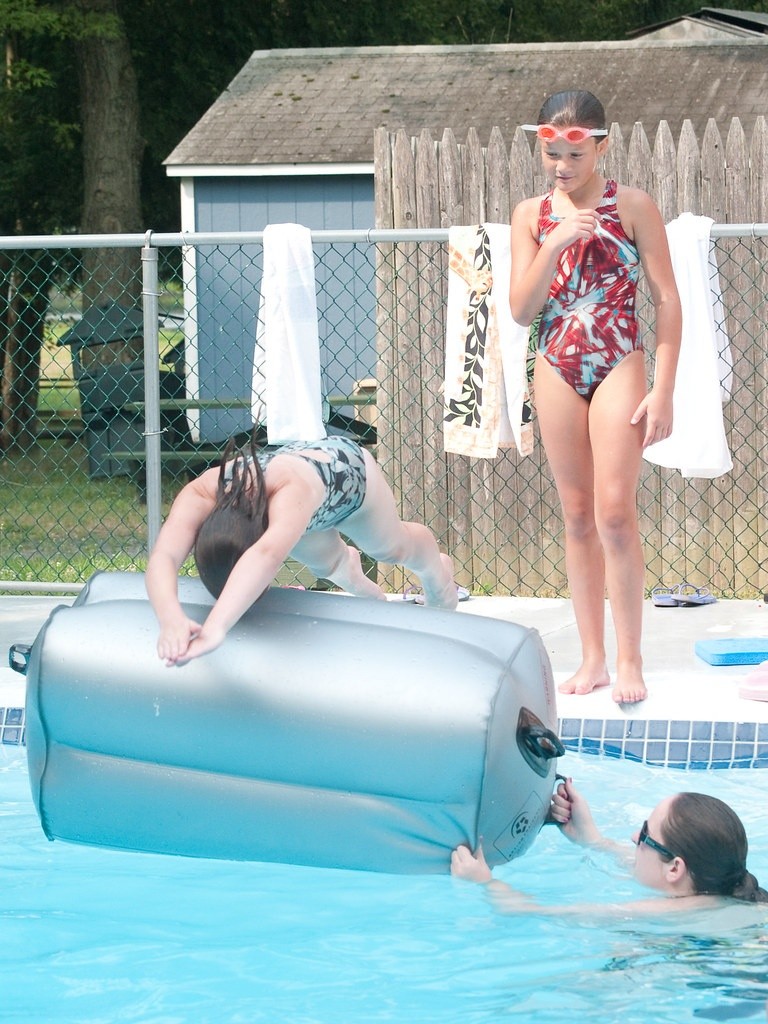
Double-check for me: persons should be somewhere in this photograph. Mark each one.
[510,89,682,705]
[146,438,459,666]
[452,776,768,916]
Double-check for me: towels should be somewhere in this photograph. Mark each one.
[250,211,735,480]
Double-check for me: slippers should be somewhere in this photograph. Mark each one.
[390,586,422,603]
[416,582,470,605]
[652,585,679,606]
[671,584,717,604]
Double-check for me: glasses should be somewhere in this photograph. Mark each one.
[537,124,608,144]
[638,820,676,861]
